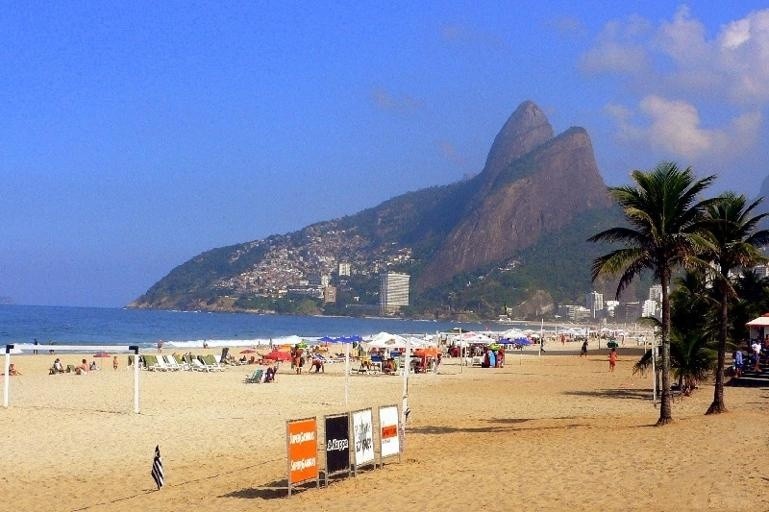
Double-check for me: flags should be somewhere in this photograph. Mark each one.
[151,445,164,490]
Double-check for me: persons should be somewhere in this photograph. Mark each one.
[9,363,18,376]
[203,339,207,348]
[607,348,618,371]
[77,358,89,374]
[53,356,61,371]
[732,333,769,379]
[223,343,504,384]
[34,338,39,354]
[182,352,188,364]
[56,358,64,369]
[157,340,163,353]
[113,356,118,369]
[47,337,56,354]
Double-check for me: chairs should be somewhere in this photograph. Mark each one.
[129,350,225,373]
[242,365,278,383]
